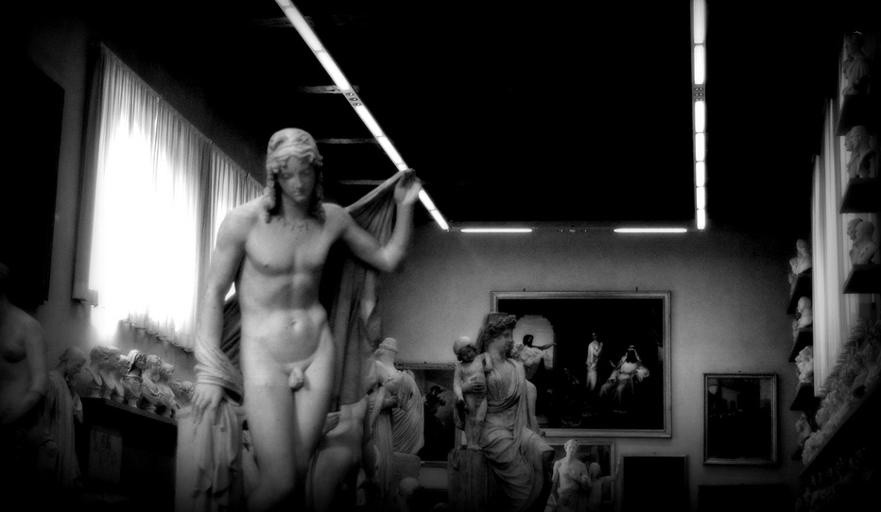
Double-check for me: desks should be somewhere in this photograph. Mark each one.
[80,397,176,512]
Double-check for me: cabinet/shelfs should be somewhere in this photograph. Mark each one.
[835,94,879,293]
[787,268,813,459]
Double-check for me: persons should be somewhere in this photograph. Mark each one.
[190,125,421,512]
[787,40,881,467]
[0,261,649,512]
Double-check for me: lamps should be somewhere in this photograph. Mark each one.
[691,0,708,229]
[449,225,688,234]
[275,0,450,234]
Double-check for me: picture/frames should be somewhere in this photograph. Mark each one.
[702,373,778,467]
[548,442,616,512]
[491,291,672,438]
[393,362,462,468]
[619,453,690,512]
